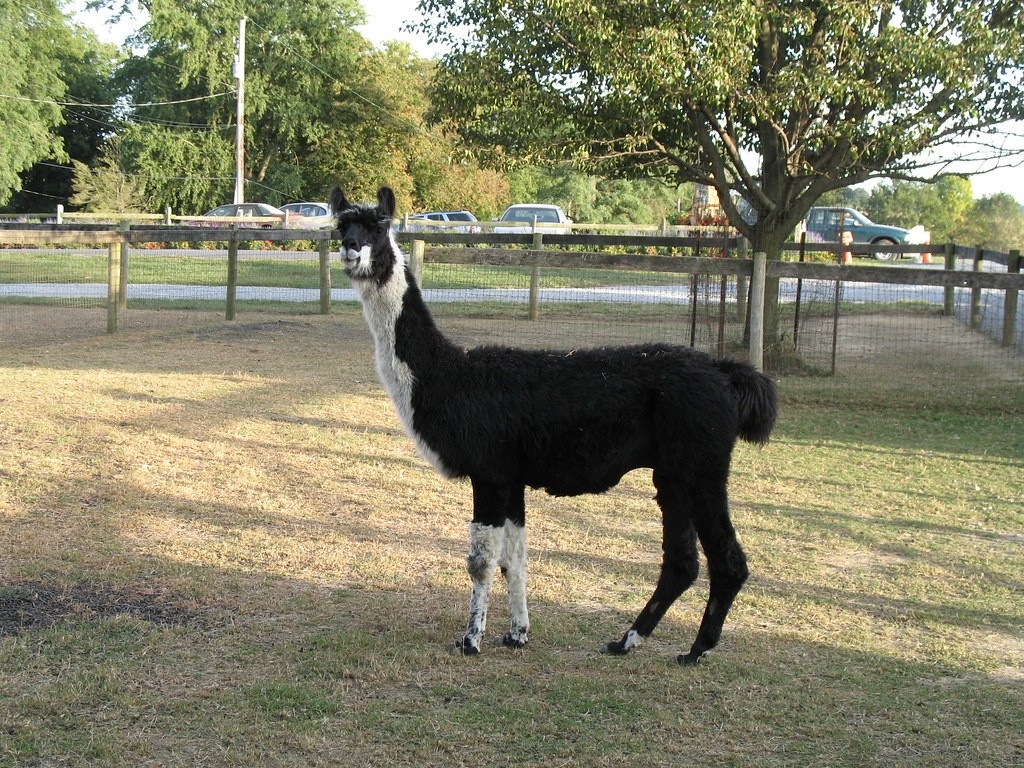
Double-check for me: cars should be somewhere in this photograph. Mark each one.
[491,203,572,234]
[275,203,338,231]
[184,201,305,245]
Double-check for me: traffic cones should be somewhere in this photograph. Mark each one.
[922,241,935,266]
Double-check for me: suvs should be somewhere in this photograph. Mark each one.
[400,209,483,234]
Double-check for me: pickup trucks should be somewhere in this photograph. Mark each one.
[804,206,913,263]
